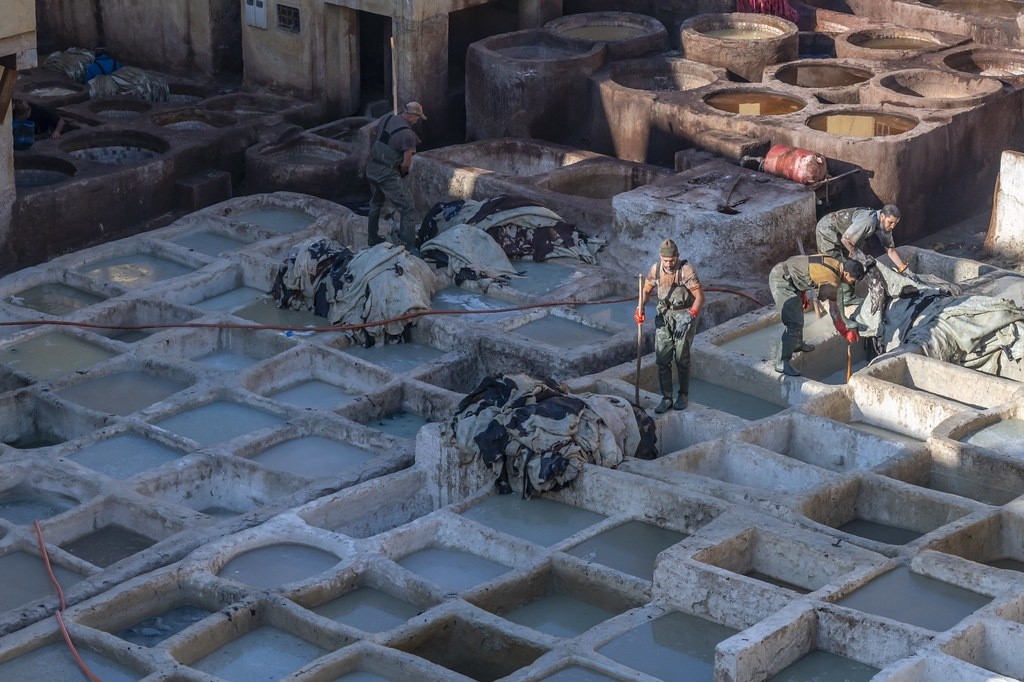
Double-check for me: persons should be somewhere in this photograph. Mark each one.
[13,102,65,140]
[634,239,704,414]
[768,254,864,376]
[816,205,909,328]
[363,101,427,260]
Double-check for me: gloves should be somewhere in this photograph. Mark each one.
[673,312,695,340]
[800,290,810,309]
[833,319,859,343]
[634,307,645,323]
[899,265,910,277]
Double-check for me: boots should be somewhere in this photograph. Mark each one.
[400,221,418,256]
[368,214,386,246]
[655,369,673,414]
[775,331,801,376]
[673,367,690,410]
[793,327,815,352]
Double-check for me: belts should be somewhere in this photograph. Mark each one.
[371,159,394,169]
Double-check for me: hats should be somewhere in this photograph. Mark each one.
[403,102,427,120]
[658,239,678,258]
